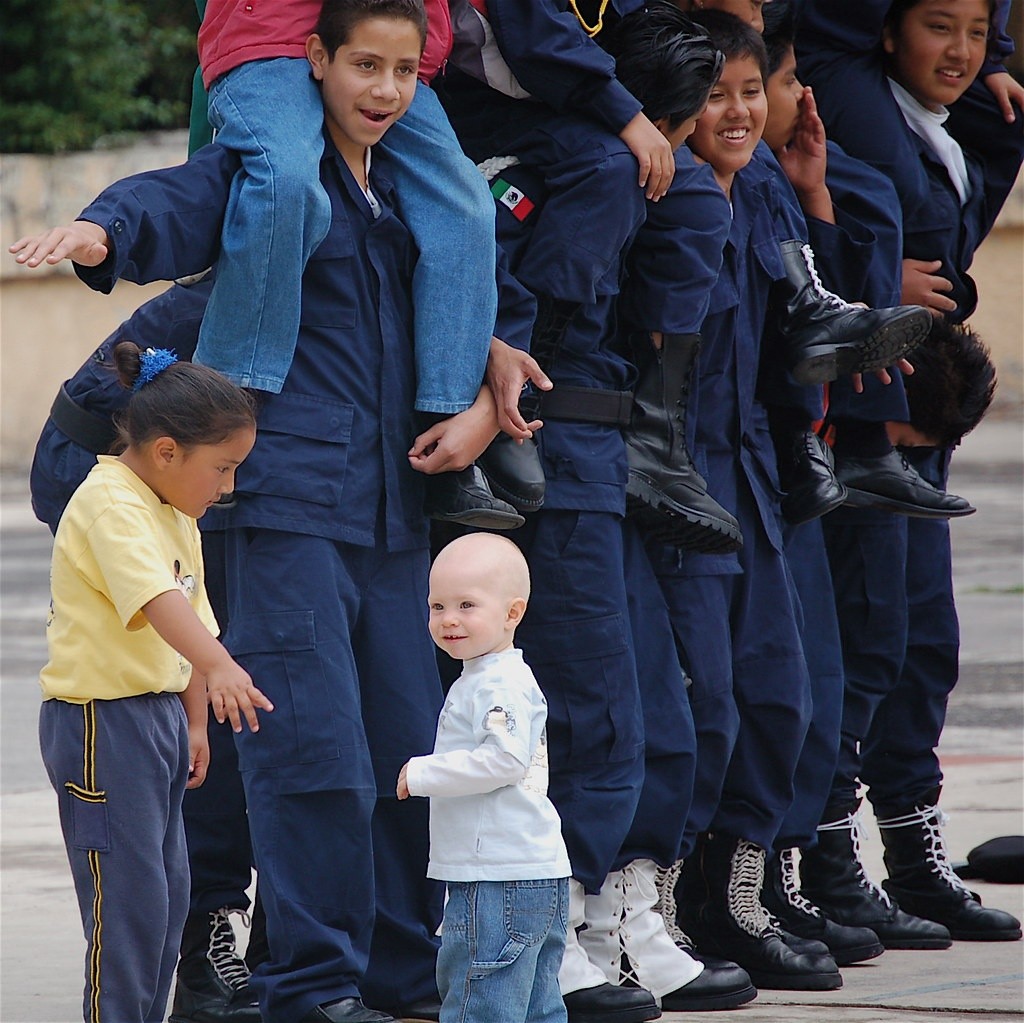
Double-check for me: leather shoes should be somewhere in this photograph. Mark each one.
[562,983,660,1023]
[299,997,401,1023]
[776,429,848,527]
[383,999,442,1022]
[661,967,757,1011]
[834,447,976,518]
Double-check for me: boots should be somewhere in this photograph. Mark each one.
[875,786,1022,940]
[624,333,742,555]
[758,841,884,965]
[696,832,842,989]
[211,388,270,508]
[168,905,265,1023]
[425,413,524,529]
[655,859,739,969]
[753,888,836,966]
[766,239,933,385]
[478,285,584,513]
[800,797,952,951]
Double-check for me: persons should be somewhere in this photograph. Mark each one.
[394,531,572,1023]
[7,0,1024,1023]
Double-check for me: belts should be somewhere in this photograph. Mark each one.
[50,379,129,456]
[539,383,633,425]
